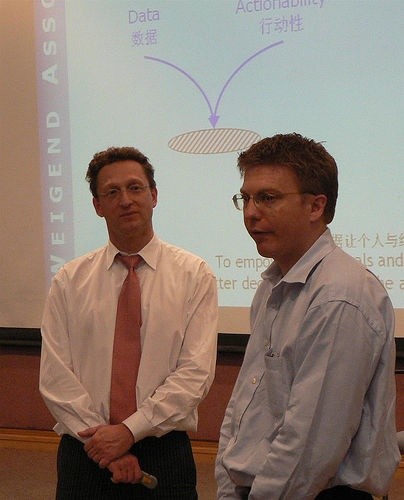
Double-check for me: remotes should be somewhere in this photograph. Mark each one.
[140,470,158,489]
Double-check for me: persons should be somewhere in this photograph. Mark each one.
[39,146,218,500]
[214,131,402,500]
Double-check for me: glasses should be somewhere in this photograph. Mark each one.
[231,191,318,210]
[96,183,154,201]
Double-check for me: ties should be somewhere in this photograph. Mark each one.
[108,255,147,426]
